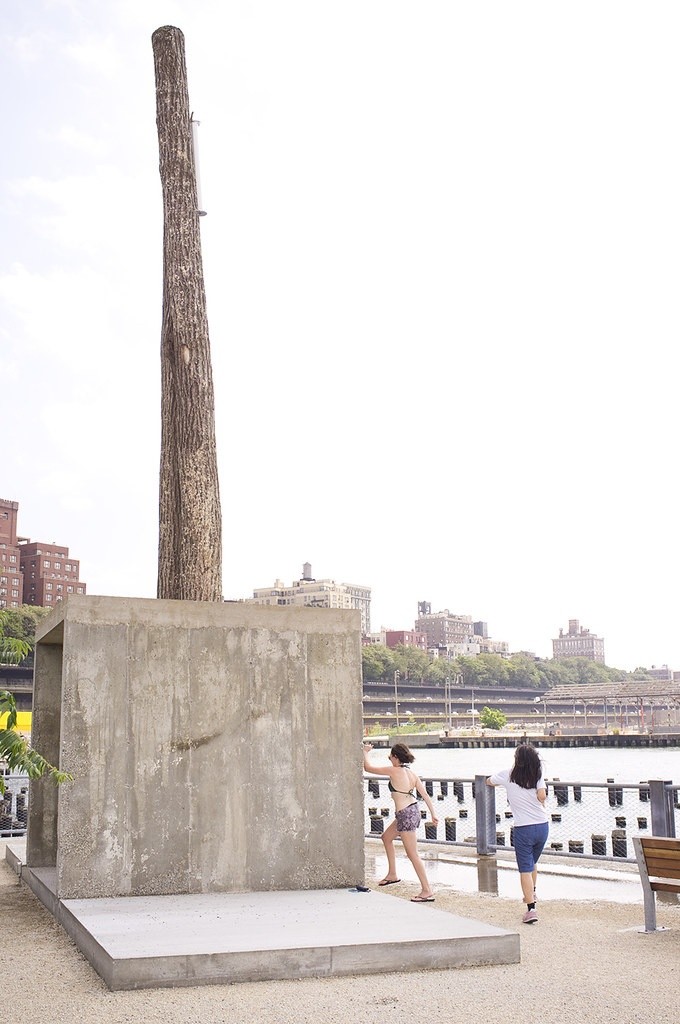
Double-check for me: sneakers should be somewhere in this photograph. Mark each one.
[522,908,539,922]
[523,892,537,903]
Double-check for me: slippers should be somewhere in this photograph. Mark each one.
[378,879,401,886]
[411,895,435,902]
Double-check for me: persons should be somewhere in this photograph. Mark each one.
[486,745,549,922]
[0,784,29,837]
[363,743,438,902]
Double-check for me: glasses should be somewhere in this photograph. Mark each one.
[388,757,393,760]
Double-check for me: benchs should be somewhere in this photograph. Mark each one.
[632,835,680,933]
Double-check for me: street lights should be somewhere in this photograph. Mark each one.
[395,670,400,726]
[446,677,452,725]
